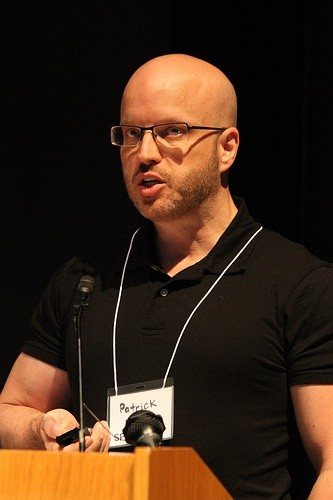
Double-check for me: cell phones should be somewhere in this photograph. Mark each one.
[56,426,84,448]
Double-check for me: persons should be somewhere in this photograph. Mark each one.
[0,54,333,500]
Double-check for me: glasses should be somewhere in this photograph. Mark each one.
[111,121,223,150]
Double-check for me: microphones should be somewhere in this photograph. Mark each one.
[75,277,88,452]
[123,409,166,448]
